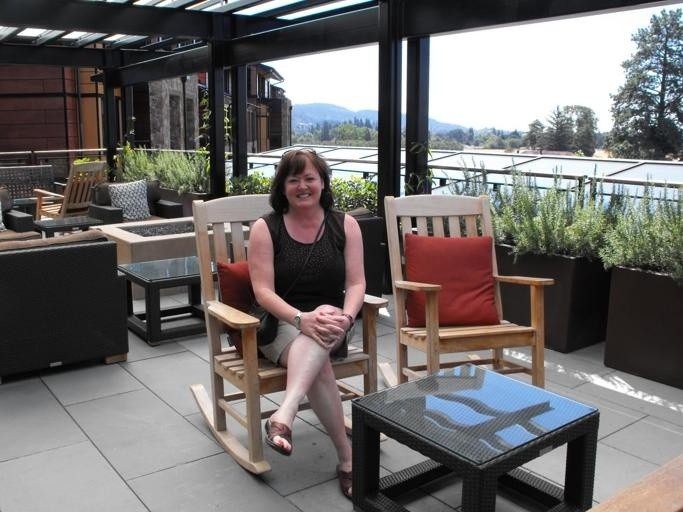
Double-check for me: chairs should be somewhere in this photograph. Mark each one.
[0,187,41,242]
[384,194,555,389]
[0,230,129,383]
[32,161,106,236]
[89,180,183,225]
[190,193,388,475]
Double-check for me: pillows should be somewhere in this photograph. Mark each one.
[58,177,95,213]
[405,233,500,328]
[107,179,152,223]
[217,262,255,359]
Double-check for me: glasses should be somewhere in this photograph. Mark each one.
[281,148,317,162]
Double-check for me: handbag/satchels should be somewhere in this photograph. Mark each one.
[239,303,277,347]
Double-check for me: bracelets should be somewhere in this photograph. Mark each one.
[341,313,353,328]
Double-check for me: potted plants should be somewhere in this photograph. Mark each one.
[599,173,683,391]
[496,157,625,354]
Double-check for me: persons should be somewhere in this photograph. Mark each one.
[239,148,366,498]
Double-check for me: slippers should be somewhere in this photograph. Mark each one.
[336,464,352,500]
[265,419,293,456]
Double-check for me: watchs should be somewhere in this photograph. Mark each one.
[294,311,301,330]
